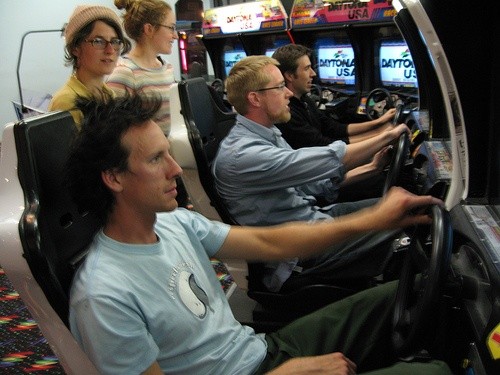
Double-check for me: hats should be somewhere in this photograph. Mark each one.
[66,4,124,56]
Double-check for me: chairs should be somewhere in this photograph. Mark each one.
[0,108,101,375]
[178,77,294,308]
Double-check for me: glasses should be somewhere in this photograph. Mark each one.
[82,36,121,49]
[157,24,174,32]
[255,83,286,91]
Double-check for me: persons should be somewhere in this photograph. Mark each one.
[49,4,132,135]
[105,0,195,212]
[68,86,451,375]
[213,43,413,293]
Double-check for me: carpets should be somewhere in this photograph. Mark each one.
[1,191,237,375]
